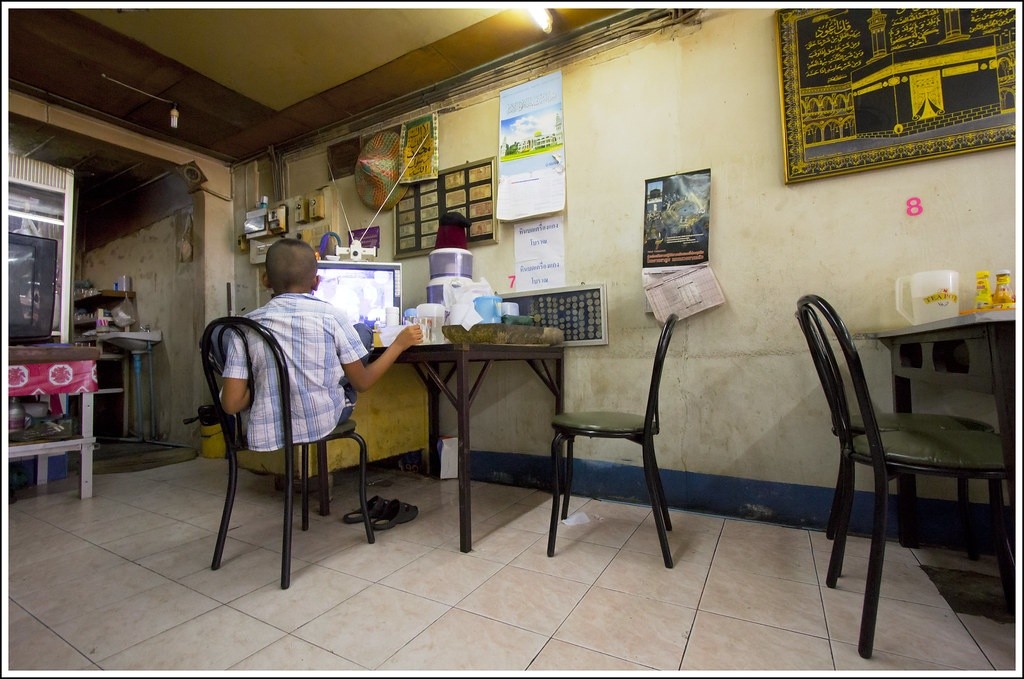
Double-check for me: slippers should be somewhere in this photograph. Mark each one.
[370,498,418,530]
[344,496,392,523]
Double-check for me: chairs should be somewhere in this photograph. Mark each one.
[795,294,1015,660]
[199,316,374,590]
[546,314,679,569]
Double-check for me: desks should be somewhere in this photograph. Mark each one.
[316,343,564,552]
[851,307,1015,549]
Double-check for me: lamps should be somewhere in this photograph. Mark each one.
[528,8,553,35]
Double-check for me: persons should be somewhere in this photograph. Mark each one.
[221,238,423,452]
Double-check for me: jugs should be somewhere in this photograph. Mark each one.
[896,269,959,325]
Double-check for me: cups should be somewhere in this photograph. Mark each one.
[386,306,400,327]
[403,308,416,325]
[473,296,503,324]
[416,303,446,344]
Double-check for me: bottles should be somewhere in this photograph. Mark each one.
[991,270,1016,308]
[975,270,994,310]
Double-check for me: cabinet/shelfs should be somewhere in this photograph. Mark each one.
[73,290,136,439]
[9,346,102,500]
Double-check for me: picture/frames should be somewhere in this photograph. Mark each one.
[391,156,501,262]
[498,282,609,347]
[775,8,1015,185]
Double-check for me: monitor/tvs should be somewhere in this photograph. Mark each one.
[313,261,402,329]
[7,232,58,346]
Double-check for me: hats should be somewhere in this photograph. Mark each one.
[354,131,409,211]
